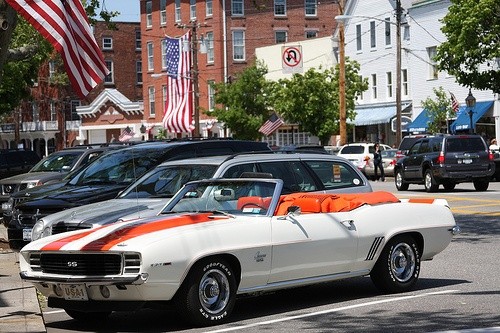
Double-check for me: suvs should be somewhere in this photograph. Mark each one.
[276,142,333,183]
[0,139,147,228]
[7,135,277,252]
[338,142,391,174]
[396,134,435,161]
[392,132,497,192]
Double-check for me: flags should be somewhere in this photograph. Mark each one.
[6,0,112,101]
[161,29,194,133]
[257,111,283,136]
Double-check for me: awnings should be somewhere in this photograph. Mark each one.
[406,100,494,132]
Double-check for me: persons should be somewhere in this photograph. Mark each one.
[489,138,500,156]
[374,142,386,183]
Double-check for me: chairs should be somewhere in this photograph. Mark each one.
[292,197,322,214]
[237,197,264,210]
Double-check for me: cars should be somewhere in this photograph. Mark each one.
[30,151,375,242]
[324,145,344,157]
[363,148,401,181]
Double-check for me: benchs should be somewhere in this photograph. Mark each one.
[0,151,34,171]
[276,195,361,215]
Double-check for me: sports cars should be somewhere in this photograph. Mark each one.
[16,170,461,328]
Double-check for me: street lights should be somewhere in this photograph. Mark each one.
[150,71,200,137]
[333,13,402,150]
